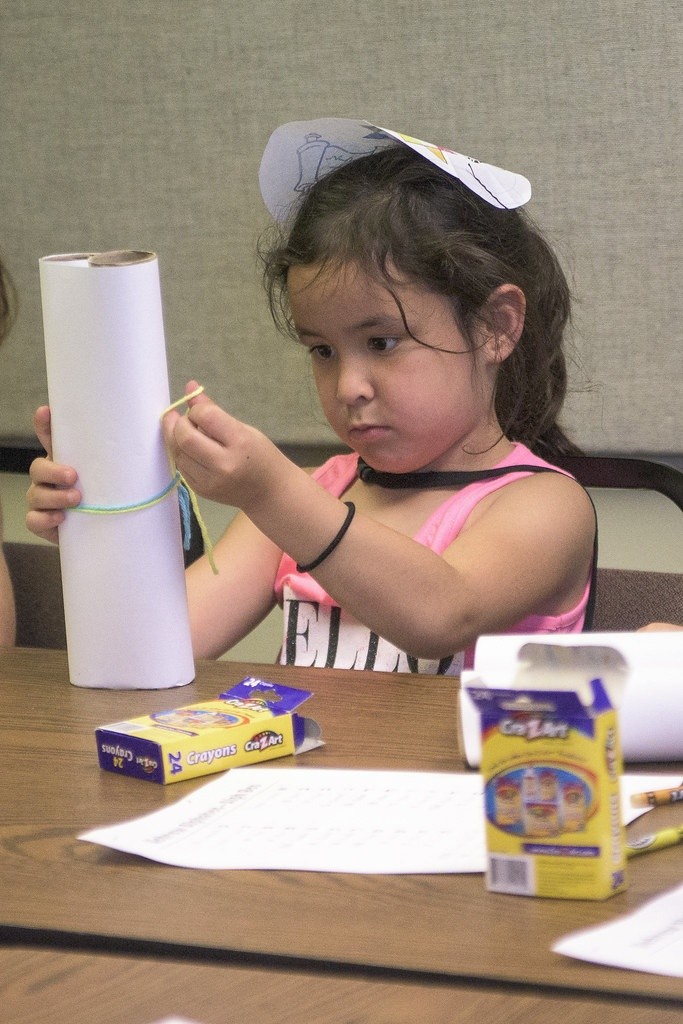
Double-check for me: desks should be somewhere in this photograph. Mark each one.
[0,658,683,1024]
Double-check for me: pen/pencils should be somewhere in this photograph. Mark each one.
[625,827,683,858]
[631,785,683,808]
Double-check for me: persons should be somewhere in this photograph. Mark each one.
[0,258,20,645]
[30,117,597,677]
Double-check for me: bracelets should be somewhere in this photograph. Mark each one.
[297,501,355,573]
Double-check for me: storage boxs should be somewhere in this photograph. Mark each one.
[95,676,325,787]
[464,640,627,899]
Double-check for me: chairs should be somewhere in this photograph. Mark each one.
[571,456,683,635]
